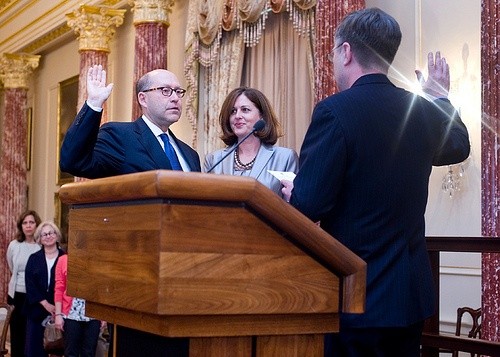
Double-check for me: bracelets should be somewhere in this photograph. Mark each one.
[53,313,64,317]
[48,307,56,314]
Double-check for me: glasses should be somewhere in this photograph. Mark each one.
[40,231,57,238]
[141,86,187,100]
[327,42,344,64]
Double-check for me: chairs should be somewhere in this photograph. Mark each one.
[0,303,15,357]
[439,306,482,357]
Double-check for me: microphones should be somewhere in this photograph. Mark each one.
[206,119,266,173]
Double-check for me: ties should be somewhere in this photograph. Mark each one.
[156,132,183,170]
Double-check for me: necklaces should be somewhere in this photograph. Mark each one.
[234,149,256,171]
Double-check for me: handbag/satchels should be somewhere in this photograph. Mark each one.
[40,313,69,352]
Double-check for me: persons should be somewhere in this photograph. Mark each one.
[203,87,299,203]
[25,223,64,357]
[5,210,43,357]
[54,253,101,357]
[281,7,471,357]
[60,64,201,357]
